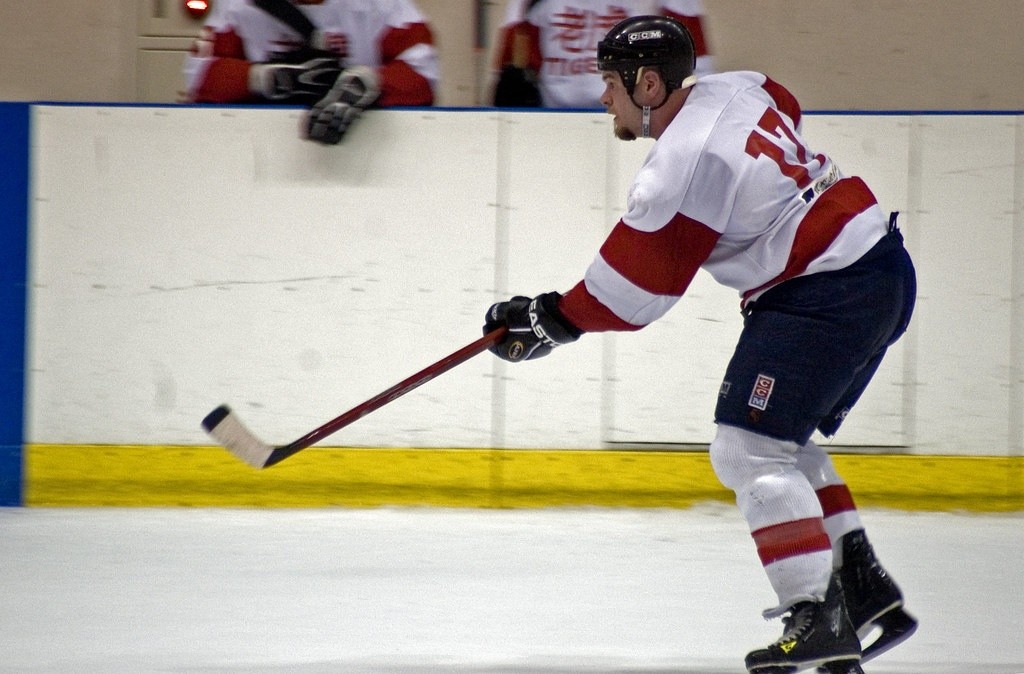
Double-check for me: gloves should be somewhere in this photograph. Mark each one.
[483,290,586,362]
[306,74,368,144]
[255,56,341,97]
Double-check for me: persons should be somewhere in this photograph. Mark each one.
[486,0,713,109]
[483,15,916,674]
[175,0,440,146]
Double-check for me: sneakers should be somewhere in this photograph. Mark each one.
[833,527,919,664]
[746,571,863,674]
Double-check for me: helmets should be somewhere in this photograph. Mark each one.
[596,15,695,88]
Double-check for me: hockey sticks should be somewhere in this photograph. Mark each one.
[200,324,509,468]
